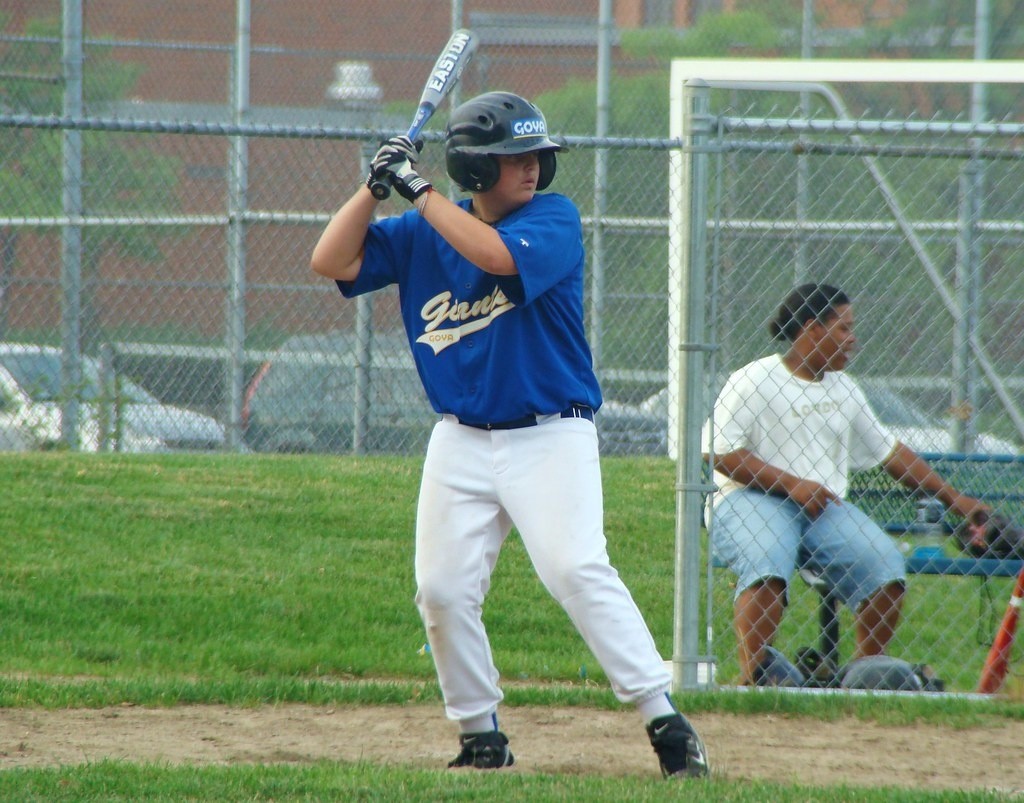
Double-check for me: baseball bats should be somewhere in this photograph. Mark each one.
[976,568,1024,692]
[369,28,481,201]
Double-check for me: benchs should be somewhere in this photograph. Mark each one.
[708,456,1024,672]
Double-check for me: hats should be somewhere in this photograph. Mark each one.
[446,90,571,192]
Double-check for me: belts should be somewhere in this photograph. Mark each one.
[459,402,594,431]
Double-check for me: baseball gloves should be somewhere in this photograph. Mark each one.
[955,507,1023,558]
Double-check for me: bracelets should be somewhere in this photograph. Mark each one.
[419,189,437,215]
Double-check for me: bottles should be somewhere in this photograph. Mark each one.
[913,488,945,560]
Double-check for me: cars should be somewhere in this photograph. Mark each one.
[640,370,1020,454]
[0,343,241,455]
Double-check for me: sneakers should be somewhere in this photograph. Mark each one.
[646,711,708,779]
[447,731,515,769]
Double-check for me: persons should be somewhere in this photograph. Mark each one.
[700,285,992,685]
[311,89,710,778]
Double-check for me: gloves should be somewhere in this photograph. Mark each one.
[365,135,432,202]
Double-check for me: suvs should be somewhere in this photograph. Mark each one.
[240,331,668,455]
